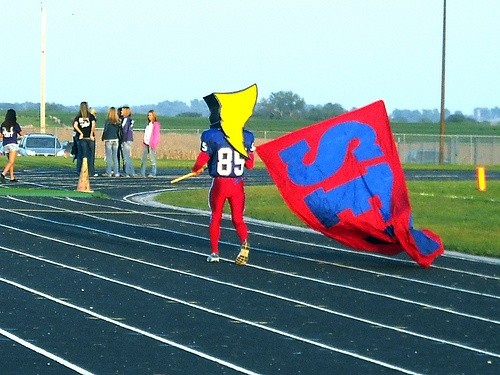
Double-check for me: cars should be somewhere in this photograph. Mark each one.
[0,142,21,156]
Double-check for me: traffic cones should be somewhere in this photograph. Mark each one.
[74,157,94,193]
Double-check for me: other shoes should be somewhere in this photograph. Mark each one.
[10,179,18,182]
[101,173,111,177]
[113,174,119,177]
[207,253,219,262]
[236,242,249,266]
[0,174,6,183]
[94,173,98,176]
[148,175,156,177]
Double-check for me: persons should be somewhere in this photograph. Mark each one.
[101,106,134,178]
[0,109,26,184]
[72,102,99,177]
[192,83,258,266]
[136,109,160,178]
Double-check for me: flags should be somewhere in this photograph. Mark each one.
[256,100,444,268]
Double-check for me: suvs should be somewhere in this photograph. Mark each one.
[62,141,76,158]
[19,132,67,158]
